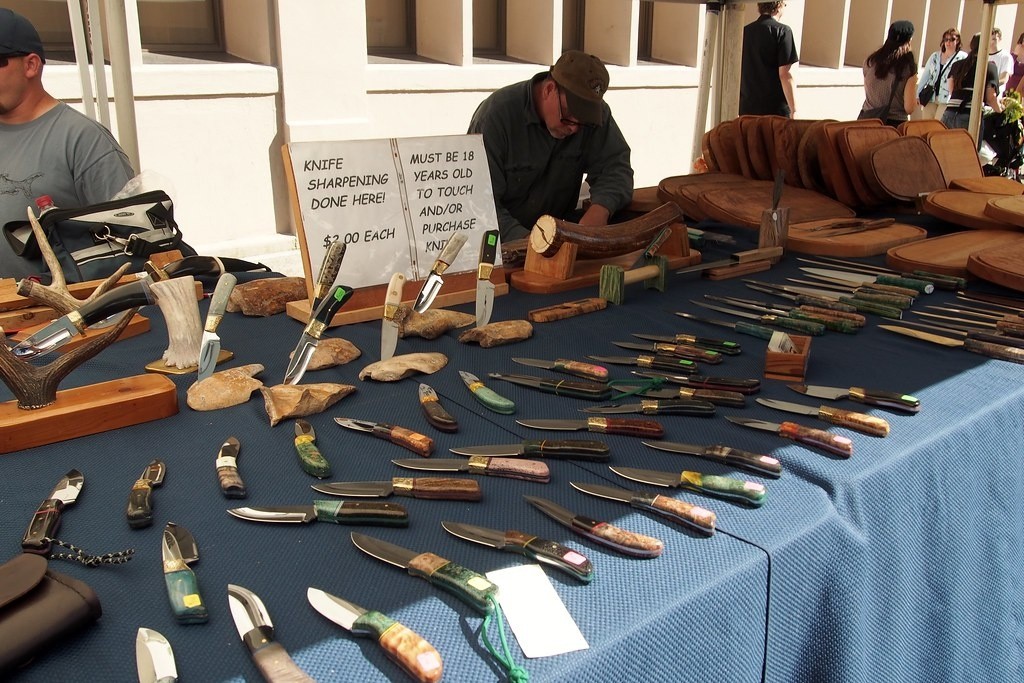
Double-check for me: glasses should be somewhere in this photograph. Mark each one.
[943,38,957,42]
[0,53,45,67]
[555,82,596,128]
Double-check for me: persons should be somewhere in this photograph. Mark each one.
[941,31,1003,132]
[738,0,801,121]
[916,27,968,121]
[0,8,137,285]
[987,27,1014,99]
[468,50,635,247]
[857,21,921,129]
[1002,33,1024,185]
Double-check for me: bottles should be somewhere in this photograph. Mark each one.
[34,194,59,220]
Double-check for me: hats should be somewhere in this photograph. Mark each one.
[0,8,45,61]
[551,50,609,128]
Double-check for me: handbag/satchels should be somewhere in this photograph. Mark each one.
[857,106,890,126]
[3,190,197,288]
[919,85,934,106]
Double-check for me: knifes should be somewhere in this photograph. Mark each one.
[9,218,1023,682]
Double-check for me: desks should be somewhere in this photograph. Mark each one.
[0,254,1023,683]
